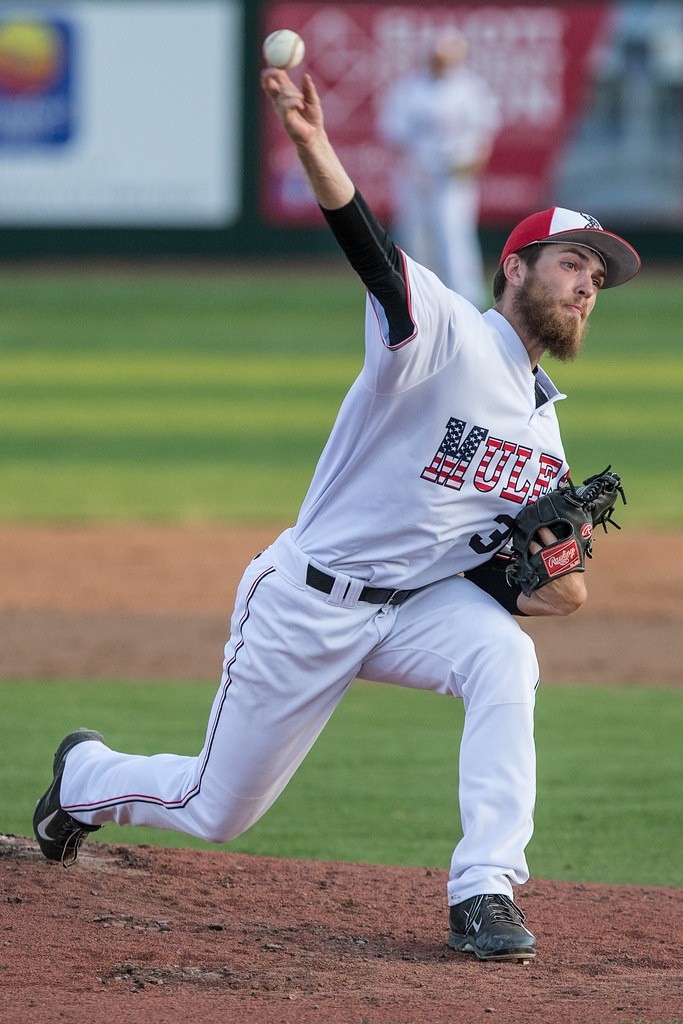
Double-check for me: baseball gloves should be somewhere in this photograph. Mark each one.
[508,470,620,599]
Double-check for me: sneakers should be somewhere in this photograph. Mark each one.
[33,730,105,862]
[448,894,539,959]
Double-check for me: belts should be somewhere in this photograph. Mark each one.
[306,562,412,605]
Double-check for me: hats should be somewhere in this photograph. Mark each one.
[499,206,640,289]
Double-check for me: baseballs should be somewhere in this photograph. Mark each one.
[262,29,306,70]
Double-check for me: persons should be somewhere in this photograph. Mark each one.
[381,28,500,311]
[31,68,640,965]
